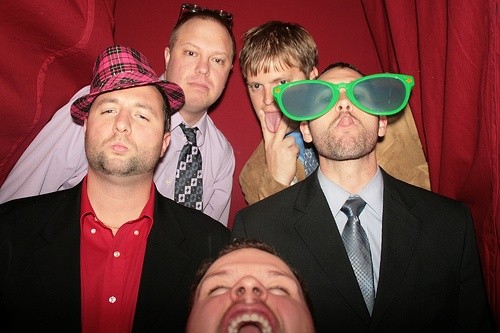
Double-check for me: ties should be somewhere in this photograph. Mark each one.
[304,143,320,177]
[174,122,203,212]
[340,197,375,316]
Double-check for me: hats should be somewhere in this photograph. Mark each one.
[70,45,186,126]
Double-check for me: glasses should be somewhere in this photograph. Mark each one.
[272,73,414,120]
[178,2,233,29]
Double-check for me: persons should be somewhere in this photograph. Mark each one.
[0,11,236,228]
[231,63,488,333]
[238,20,431,205]
[0,45,232,333]
[185,236,316,333]
